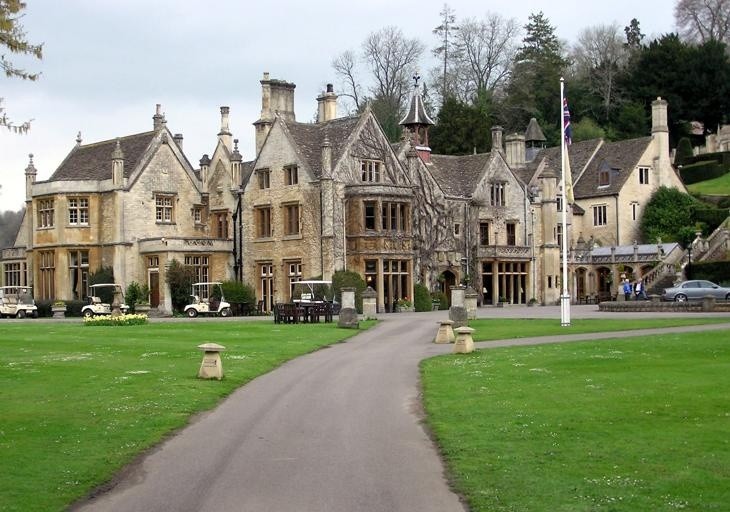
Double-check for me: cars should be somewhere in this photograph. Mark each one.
[662,280,730,302]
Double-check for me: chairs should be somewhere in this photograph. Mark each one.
[272,299,333,324]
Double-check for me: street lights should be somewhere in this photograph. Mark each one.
[686,237,692,270]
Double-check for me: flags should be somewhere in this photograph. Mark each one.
[565,141,577,206]
[564,95,576,145]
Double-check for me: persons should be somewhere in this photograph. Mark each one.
[482,284,490,304]
[635,278,651,300]
[623,278,634,299]
